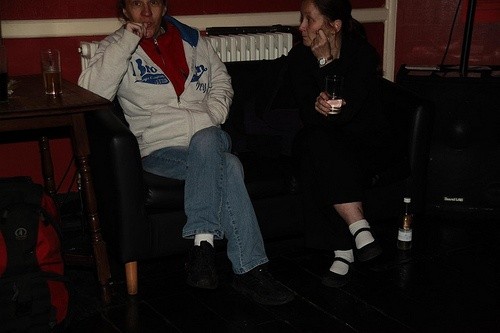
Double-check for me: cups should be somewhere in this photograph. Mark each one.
[41,47,63,95]
[325,74,343,114]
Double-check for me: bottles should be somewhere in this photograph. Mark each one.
[397,198,415,250]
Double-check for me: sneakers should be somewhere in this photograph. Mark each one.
[184,241,220,289]
[235,265,295,304]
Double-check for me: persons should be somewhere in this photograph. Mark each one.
[74,0,296,308]
[278,0,402,289]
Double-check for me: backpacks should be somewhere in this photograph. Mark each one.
[0,177,72,333]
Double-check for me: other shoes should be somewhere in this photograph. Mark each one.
[353,228,382,262]
[316,257,353,293]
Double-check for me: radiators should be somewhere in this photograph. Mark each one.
[78,30,293,73]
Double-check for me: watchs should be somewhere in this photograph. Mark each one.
[317,54,333,66]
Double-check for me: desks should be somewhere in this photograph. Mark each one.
[395,64,500,243]
[1,72,114,312]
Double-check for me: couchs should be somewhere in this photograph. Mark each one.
[83,79,436,295]
